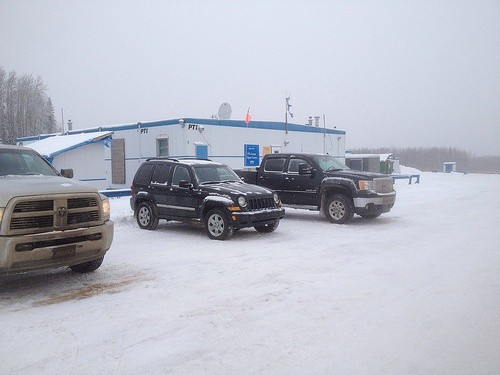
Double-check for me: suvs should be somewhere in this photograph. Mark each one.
[129,156,286,240]
[0,141,114,273]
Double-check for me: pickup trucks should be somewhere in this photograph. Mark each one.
[230,149,396,224]
